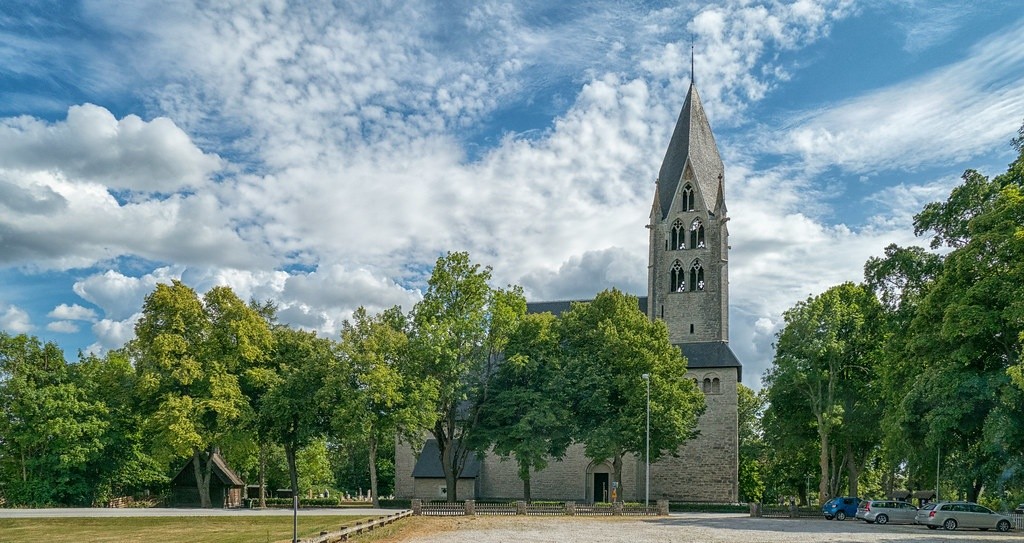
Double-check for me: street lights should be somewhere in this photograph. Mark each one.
[641,373,650,516]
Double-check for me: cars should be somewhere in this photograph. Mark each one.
[1015,503,1024,516]
[822,496,863,522]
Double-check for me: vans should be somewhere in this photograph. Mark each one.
[915,501,1016,533]
[855,499,919,524]
